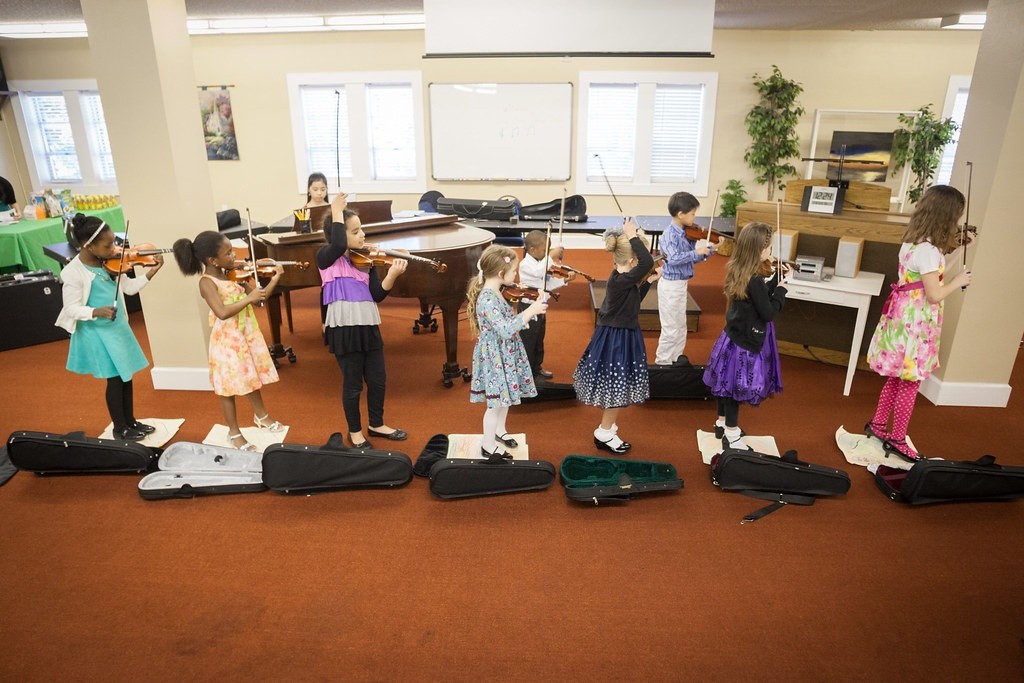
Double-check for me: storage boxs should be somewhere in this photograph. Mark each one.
[836,236,864,278]
[772,229,798,263]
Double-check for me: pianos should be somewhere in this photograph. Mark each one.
[235,208,498,386]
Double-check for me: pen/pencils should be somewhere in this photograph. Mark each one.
[294,208,311,220]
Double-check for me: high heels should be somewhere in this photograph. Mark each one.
[722,435,754,454]
[864,418,888,442]
[593,436,631,455]
[226,430,257,453]
[713,419,745,438]
[253,412,284,432]
[882,440,919,463]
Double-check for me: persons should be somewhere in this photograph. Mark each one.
[304,173,330,207]
[467,244,549,458]
[519,229,576,380]
[573,216,663,456]
[655,192,726,367]
[0,176,21,220]
[173,230,284,452]
[865,185,972,462]
[60,213,164,440]
[702,220,793,452]
[316,192,408,452]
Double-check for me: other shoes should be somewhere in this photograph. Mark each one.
[347,431,372,452]
[480,446,513,463]
[496,432,518,448]
[535,369,553,379]
[368,425,408,440]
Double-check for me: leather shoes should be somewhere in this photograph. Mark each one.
[112,420,155,441]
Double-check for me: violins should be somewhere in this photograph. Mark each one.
[758,257,803,279]
[348,242,449,275]
[500,282,562,306]
[684,222,738,247]
[548,264,597,285]
[100,242,174,277]
[937,223,977,254]
[227,256,312,283]
[638,249,670,283]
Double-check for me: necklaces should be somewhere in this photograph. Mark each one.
[89,269,111,281]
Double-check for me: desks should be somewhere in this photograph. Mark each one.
[459,215,639,237]
[0,204,125,270]
[766,263,885,396]
[637,213,736,254]
